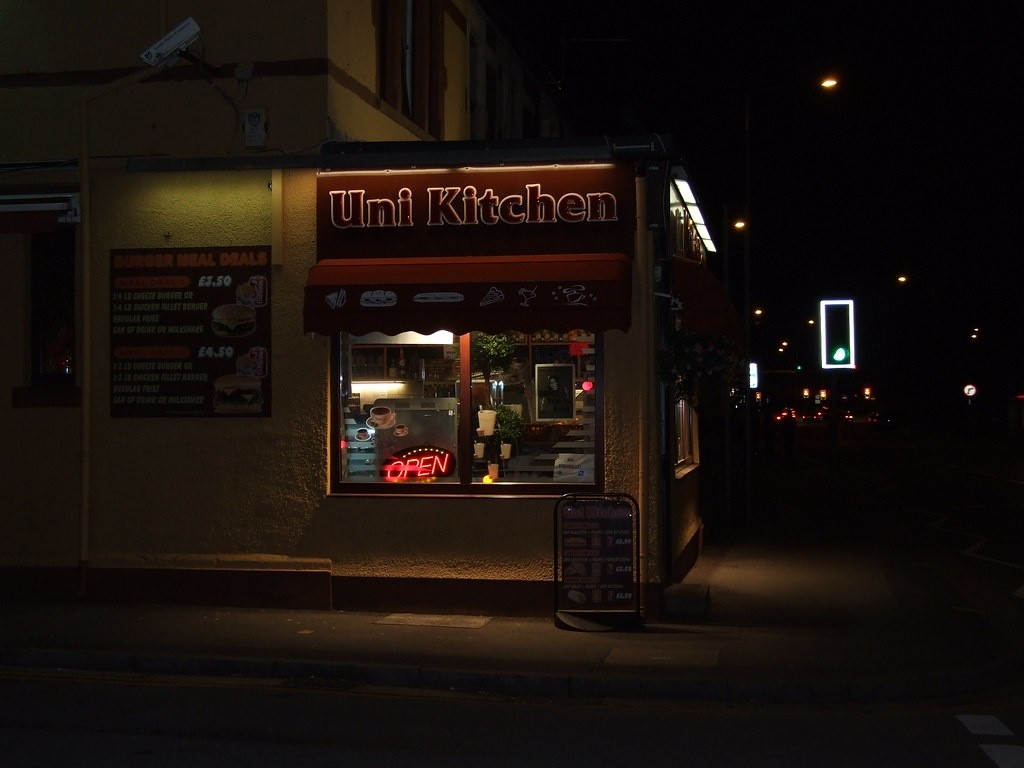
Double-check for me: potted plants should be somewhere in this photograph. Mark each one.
[475,404,527,468]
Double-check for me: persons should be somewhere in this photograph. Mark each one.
[538,375,572,415]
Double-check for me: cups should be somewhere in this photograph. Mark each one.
[356,429,370,440]
[500,444,512,459]
[395,424,408,434]
[474,443,484,458]
[370,406,396,424]
[478,410,497,436]
[488,464,498,480]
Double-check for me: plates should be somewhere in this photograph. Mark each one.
[366,417,396,429]
[355,434,371,441]
[393,430,408,437]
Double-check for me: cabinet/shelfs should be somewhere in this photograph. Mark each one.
[350,343,461,410]
